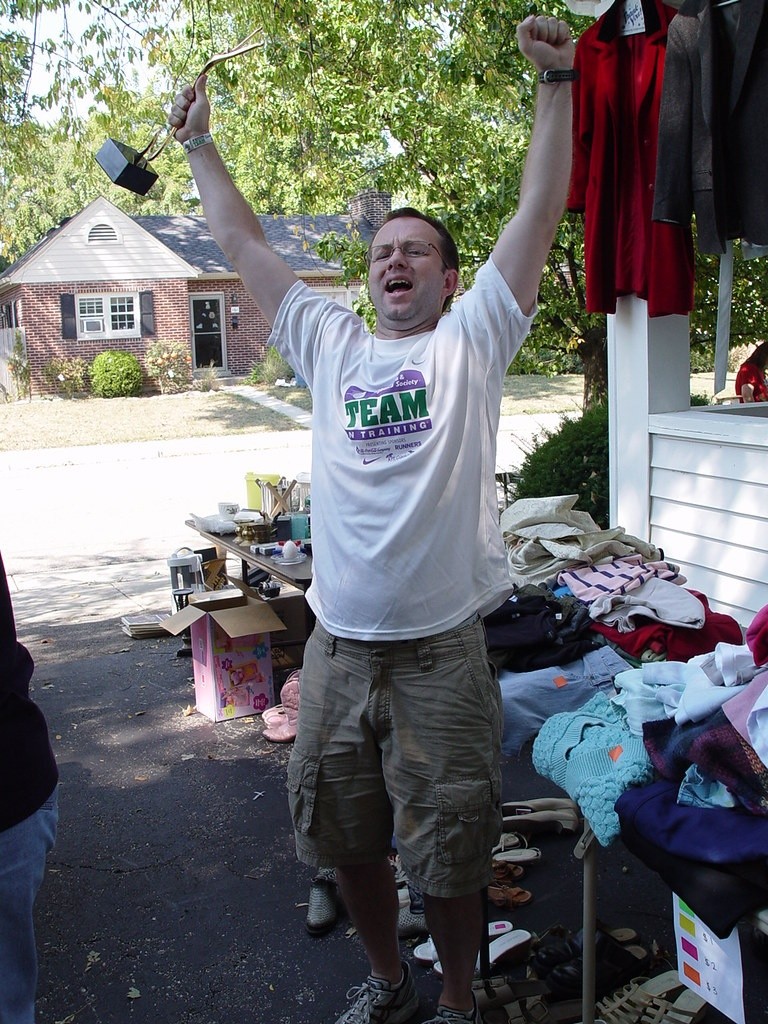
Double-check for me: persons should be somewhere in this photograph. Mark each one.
[0,551,67,1024]
[166,13,581,1023]
[735,341,768,403]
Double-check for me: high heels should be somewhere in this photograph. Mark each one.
[500,808,578,836]
[502,797,578,812]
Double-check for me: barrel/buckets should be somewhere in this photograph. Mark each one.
[245,472,280,509]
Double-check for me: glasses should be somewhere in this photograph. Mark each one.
[363,241,449,269]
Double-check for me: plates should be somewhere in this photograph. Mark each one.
[270,552,308,566]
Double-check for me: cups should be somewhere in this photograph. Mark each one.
[218,503,238,520]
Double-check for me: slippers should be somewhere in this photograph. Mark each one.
[410,914,714,1024]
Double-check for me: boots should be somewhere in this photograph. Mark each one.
[262,669,302,743]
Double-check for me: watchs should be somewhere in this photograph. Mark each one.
[536,68,577,85]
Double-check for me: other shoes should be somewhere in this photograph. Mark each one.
[386,830,541,937]
[305,877,338,935]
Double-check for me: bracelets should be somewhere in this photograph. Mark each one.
[183,133,214,154]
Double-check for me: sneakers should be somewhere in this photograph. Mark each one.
[332,960,419,1024]
[420,990,481,1024]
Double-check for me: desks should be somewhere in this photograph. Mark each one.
[495,676,768,1024]
[188,520,317,641]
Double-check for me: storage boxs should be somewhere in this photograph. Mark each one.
[159,573,288,723]
[261,591,306,673]
